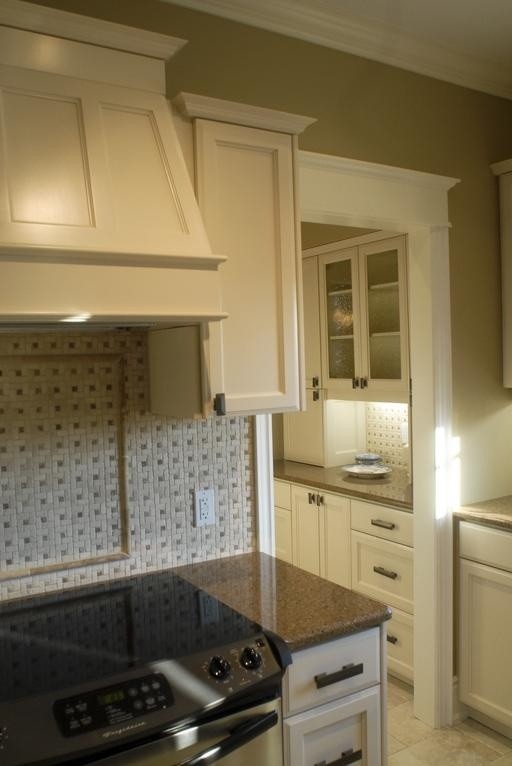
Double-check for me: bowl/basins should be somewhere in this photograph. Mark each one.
[353,452,380,463]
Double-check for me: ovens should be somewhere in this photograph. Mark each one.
[103,692,287,766]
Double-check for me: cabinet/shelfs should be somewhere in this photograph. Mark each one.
[281,621,387,765]
[450,513,512,744]
[350,498,413,689]
[291,481,351,593]
[274,479,292,565]
[318,227,409,405]
[145,92,318,419]
[282,247,366,469]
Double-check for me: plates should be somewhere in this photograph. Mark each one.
[340,463,394,480]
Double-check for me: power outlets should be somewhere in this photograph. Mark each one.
[195,490,215,528]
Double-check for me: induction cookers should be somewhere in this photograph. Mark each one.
[0,570,288,764]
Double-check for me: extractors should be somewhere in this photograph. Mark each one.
[1,34,230,334]
